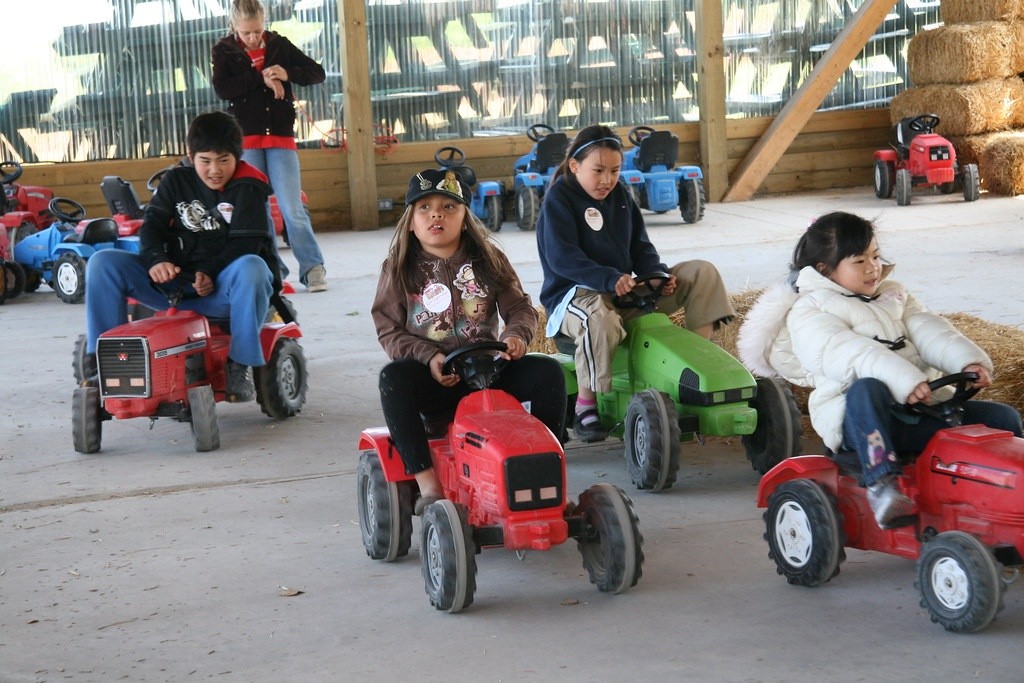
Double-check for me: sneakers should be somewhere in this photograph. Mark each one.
[867,476,913,529]
[223,356,255,403]
[307,267,327,292]
[80,374,99,386]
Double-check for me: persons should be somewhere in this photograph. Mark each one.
[371,169,568,516]
[211,0,328,292]
[736,211,1024,530]
[536,125,736,442]
[85,111,283,402]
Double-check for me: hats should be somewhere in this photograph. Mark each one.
[404,169,472,209]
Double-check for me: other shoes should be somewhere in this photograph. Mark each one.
[571,409,605,444]
[415,492,445,517]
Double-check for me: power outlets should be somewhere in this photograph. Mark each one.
[378,198,392,211]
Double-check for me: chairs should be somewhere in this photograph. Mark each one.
[531,133,573,173]
[99,176,148,217]
[63,219,119,244]
[633,131,679,171]
[450,165,476,187]
[0,183,18,214]
[896,118,930,147]
